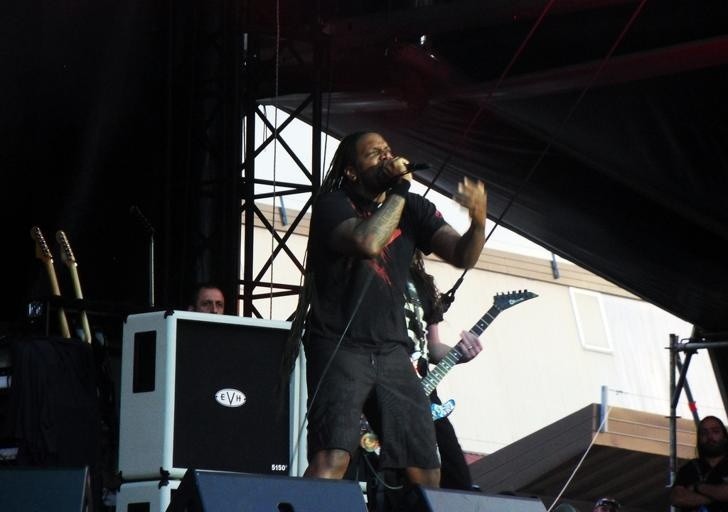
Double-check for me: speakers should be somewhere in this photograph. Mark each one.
[393,483,547,512]
[118,310,299,480]
[115,481,182,512]
[0,466,96,512]
[166,467,368,512]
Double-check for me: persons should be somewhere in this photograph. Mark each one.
[666,415,727,512]
[300,125,490,490]
[186,282,228,315]
[589,495,623,511]
[358,238,485,511]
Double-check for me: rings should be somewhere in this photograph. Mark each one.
[467,345,473,349]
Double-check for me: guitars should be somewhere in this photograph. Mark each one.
[360,290,539,454]
[56,231,92,344]
[30,225,72,340]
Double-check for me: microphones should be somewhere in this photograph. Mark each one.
[405,160,432,171]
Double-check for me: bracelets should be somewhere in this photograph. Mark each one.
[388,177,412,200]
[693,481,700,492]
